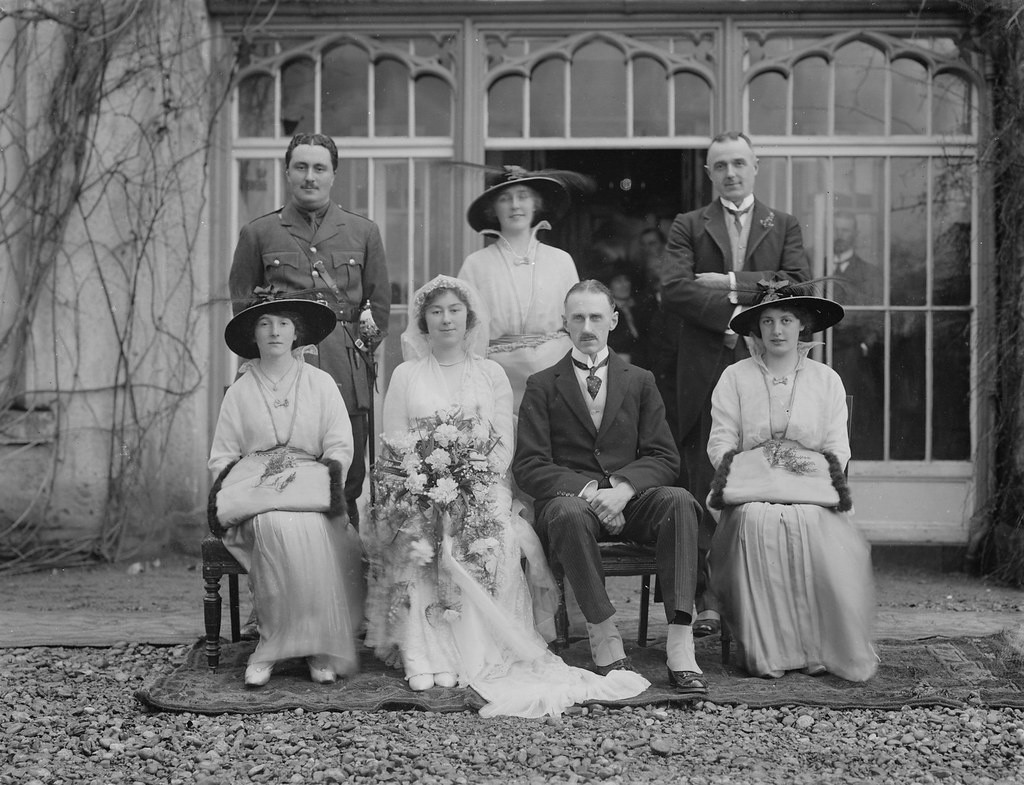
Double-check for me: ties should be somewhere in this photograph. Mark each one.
[571,355,609,400]
[834,257,852,274]
[306,211,320,232]
[718,196,755,235]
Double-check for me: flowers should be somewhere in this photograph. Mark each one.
[384,410,504,625]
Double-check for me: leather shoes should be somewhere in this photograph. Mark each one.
[595,655,638,675]
[692,619,721,638]
[668,666,709,693]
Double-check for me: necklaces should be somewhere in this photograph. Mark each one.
[258,358,295,390]
[439,359,462,368]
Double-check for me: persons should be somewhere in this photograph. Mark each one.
[448,164,581,442]
[582,212,883,430]
[706,266,880,683]
[230,134,391,535]
[215,301,366,684]
[662,130,816,640]
[374,273,652,718]
[513,283,712,695]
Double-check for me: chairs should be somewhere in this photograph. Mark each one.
[542,541,664,647]
[706,392,852,664]
[201,385,345,671]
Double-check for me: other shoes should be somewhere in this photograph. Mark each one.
[408,673,435,692]
[245,661,277,686]
[240,608,259,640]
[305,656,337,683]
[769,669,785,679]
[800,664,826,675]
[434,672,459,689]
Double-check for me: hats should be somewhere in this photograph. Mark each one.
[730,276,845,338]
[468,163,572,238]
[224,284,338,360]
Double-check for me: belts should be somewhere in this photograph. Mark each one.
[327,301,362,323]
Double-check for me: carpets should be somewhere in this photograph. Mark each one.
[132,630,1023,712]
